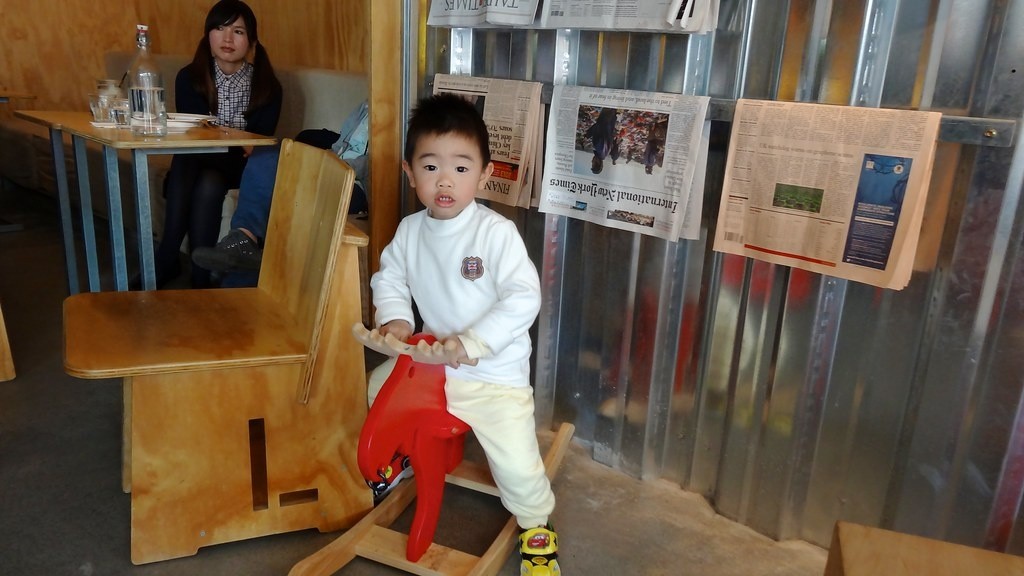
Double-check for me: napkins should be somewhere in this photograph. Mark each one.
[90,121,131,128]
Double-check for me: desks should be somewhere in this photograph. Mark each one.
[12,109,278,297]
[0,88,38,383]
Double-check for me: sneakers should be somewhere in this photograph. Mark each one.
[520,522,562,576]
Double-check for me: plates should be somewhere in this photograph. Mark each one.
[134,112,218,134]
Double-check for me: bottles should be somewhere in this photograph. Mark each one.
[128,27,168,137]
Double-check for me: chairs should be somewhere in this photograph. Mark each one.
[63,138,372,565]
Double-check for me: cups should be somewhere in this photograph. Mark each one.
[89,90,121,125]
[94,79,124,102]
[109,97,132,128]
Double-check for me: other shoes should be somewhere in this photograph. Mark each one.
[192,229,260,273]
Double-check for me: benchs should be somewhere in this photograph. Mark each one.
[0,50,370,330]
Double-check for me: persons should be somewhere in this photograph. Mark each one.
[365,92,560,576]
[157,0,283,292]
[193,98,368,289]
[583,108,623,174]
[642,140,657,175]
